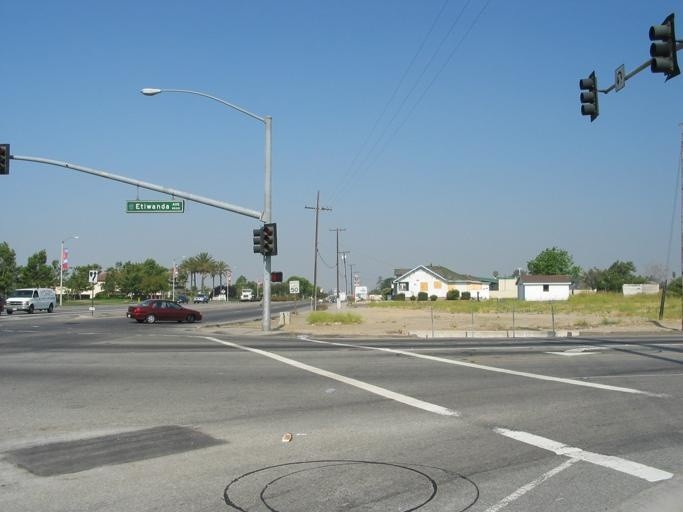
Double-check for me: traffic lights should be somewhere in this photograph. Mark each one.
[577,69,600,122]
[0,144,9,175]
[645,11,681,83]
[251,228,261,254]
[260,223,276,256]
[270,272,281,283]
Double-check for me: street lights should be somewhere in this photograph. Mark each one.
[59,232,79,306]
[139,86,272,332]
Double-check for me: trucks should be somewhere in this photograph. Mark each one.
[239,288,256,302]
[3,287,57,315]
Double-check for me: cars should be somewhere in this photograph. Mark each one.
[317,294,336,303]
[125,293,208,324]
[0,294,5,314]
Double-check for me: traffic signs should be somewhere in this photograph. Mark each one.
[289,280,299,293]
[126,200,183,213]
